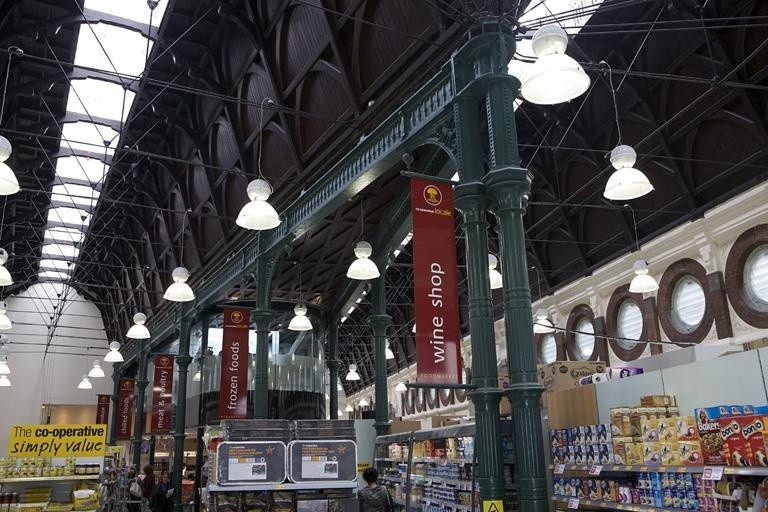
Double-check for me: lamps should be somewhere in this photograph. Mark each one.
[628,203,660,294]
[1,56,22,197]
[1,299,11,331]
[289,251,313,330]
[488,253,504,292]
[163,209,198,303]
[1,194,15,287]
[125,267,153,340]
[235,98,283,233]
[103,303,124,363]
[519,0,591,106]
[346,194,381,280]
[599,56,655,201]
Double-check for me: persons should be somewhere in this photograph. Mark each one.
[356,466,394,512]
[128,468,145,511]
[141,462,157,512]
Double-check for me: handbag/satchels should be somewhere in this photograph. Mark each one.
[130,482,143,497]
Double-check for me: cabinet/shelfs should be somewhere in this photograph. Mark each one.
[0,332,768,512]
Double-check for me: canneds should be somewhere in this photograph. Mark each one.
[0,492,18,502]
[75,463,100,474]
